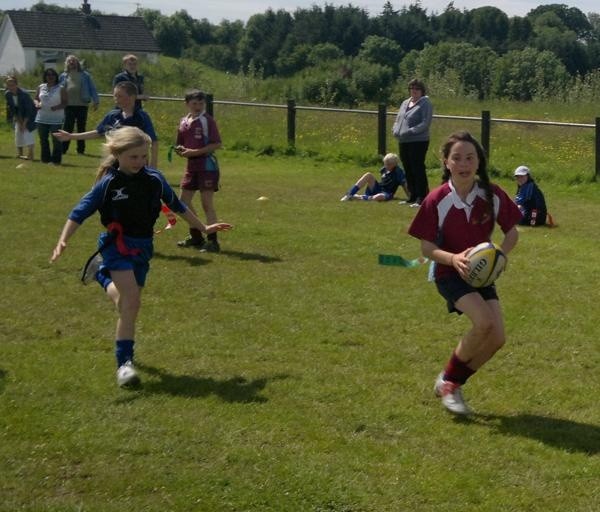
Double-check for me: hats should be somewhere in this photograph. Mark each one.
[512,164,531,176]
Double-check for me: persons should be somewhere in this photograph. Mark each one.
[46,125,235,392]
[338,153,410,203]
[3,76,36,160]
[173,89,223,255]
[58,56,101,156]
[32,68,70,166]
[511,163,549,227]
[51,81,160,170]
[392,78,432,209]
[405,132,524,418]
[113,54,148,112]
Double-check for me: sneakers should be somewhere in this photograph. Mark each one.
[409,202,423,208]
[79,252,107,287]
[339,194,353,202]
[197,240,223,255]
[431,369,468,415]
[175,232,204,248]
[353,192,365,200]
[113,358,141,390]
[13,148,88,166]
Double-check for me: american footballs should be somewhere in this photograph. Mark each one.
[463,242,507,288]
